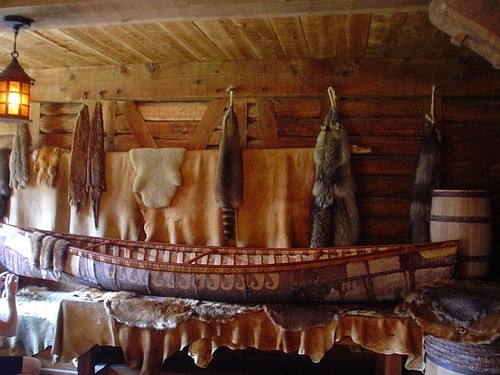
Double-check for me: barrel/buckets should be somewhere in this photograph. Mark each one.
[430,188,496,279]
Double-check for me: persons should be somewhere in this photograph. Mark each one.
[0,270,43,375]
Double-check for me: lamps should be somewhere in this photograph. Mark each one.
[0,15,34,123]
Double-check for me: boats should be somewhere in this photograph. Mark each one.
[0,222,461,304]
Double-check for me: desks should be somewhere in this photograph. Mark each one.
[0,282,499,375]
